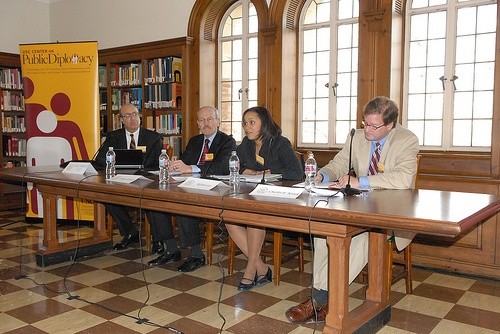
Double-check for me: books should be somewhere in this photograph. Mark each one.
[99,56,182,159]
[0,68,27,167]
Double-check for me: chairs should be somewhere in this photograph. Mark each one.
[229,151,304,286]
[107,145,213,266]
[359,154,423,303]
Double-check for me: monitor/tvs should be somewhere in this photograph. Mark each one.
[112,149,145,169]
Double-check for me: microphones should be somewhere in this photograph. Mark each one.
[200,134,232,180]
[341,128,362,196]
[135,143,157,181]
[86,134,114,171]
[256,135,274,185]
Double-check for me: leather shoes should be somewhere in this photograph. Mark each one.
[146,252,182,267]
[312,303,328,321]
[178,255,205,272]
[285,295,326,324]
[113,232,141,250]
[152,240,165,254]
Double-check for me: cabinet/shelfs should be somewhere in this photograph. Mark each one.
[0,37,195,211]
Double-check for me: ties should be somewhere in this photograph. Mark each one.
[199,139,210,165]
[129,134,136,149]
[369,142,382,175]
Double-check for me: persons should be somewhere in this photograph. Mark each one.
[285,96,420,324]
[224,107,303,290]
[147,106,236,273]
[94,103,165,254]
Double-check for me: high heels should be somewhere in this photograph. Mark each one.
[237,268,258,290]
[256,266,272,286]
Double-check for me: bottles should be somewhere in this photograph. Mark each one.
[105,147,116,174]
[229,151,240,186]
[159,149,170,181]
[304,153,317,194]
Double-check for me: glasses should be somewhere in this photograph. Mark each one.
[197,117,219,124]
[121,113,139,118]
[361,120,384,130]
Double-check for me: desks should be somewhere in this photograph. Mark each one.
[0,165,500,334]
